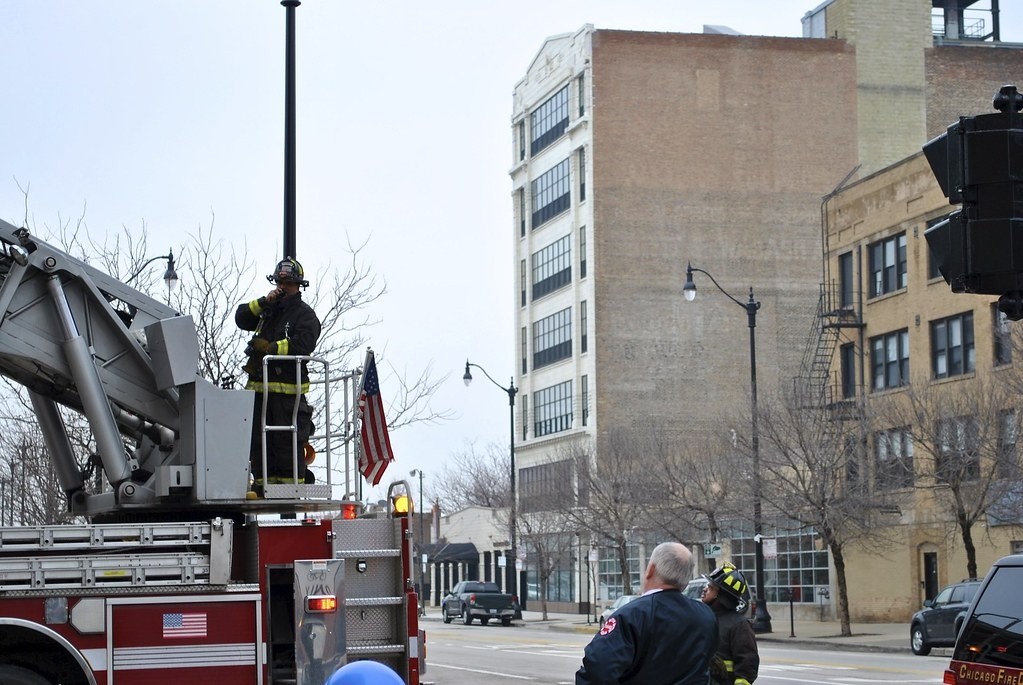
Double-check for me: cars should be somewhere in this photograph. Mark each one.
[599,595,640,628]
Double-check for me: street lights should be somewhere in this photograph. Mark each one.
[463,358,523,621]
[682,259,774,635]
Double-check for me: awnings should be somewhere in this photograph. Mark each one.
[417,543,479,563]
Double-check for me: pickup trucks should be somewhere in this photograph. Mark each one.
[441,582,519,627]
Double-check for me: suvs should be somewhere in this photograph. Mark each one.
[909,578,985,655]
[683,578,757,628]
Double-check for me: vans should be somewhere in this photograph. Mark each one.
[943,553,1023,685]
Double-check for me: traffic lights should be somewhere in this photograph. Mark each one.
[921,111,1022,296]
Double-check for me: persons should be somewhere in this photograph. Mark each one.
[701,565,760,685]
[235,256,321,500]
[574,542,720,685]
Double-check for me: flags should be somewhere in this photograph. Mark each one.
[357,355,394,487]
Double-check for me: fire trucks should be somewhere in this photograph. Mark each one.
[0,218,428,683]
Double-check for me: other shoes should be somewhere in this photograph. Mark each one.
[247,484,263,499]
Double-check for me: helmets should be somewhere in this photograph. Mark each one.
[703,564,751,605]
[273,258,304,282]
